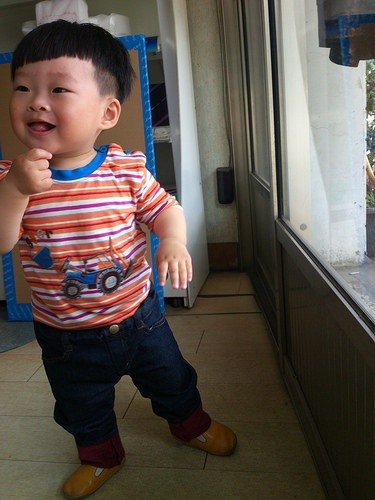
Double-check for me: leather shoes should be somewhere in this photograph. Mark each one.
[176,419,238,456]
[63,455,127,500]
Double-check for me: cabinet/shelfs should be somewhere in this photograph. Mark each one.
[0,0,212,309]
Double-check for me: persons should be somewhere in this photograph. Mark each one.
[0,19,237,500]
